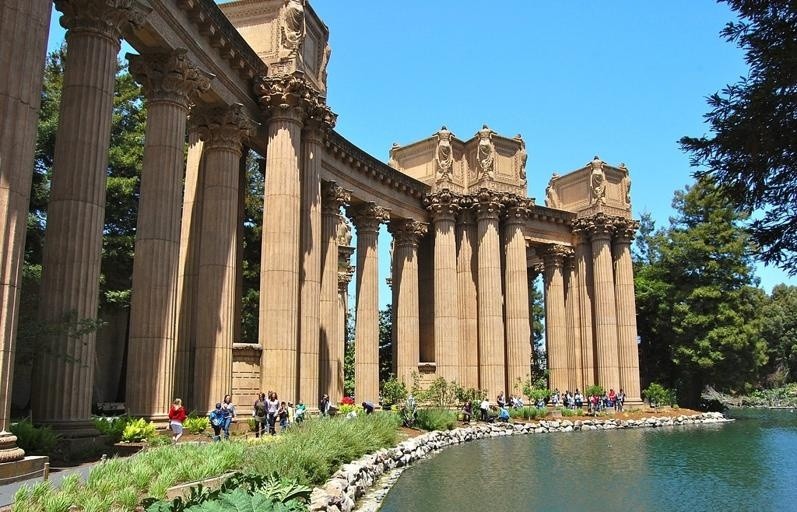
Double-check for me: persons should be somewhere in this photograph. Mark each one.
[474,124,497,180]
[512,134,528,179]
[221,394,237,441]
[585,156,607,207]
[252,388,306,438]
[209,402,229,441]
[320,393,329,416]
[432,125,455,182]
[620,161,631,203]
[389,141,400,164]
[168,398,187,443]
[546,172,559,196]
[461,387,626,424]
[284,0,308,54]
[361,402,375,415]
